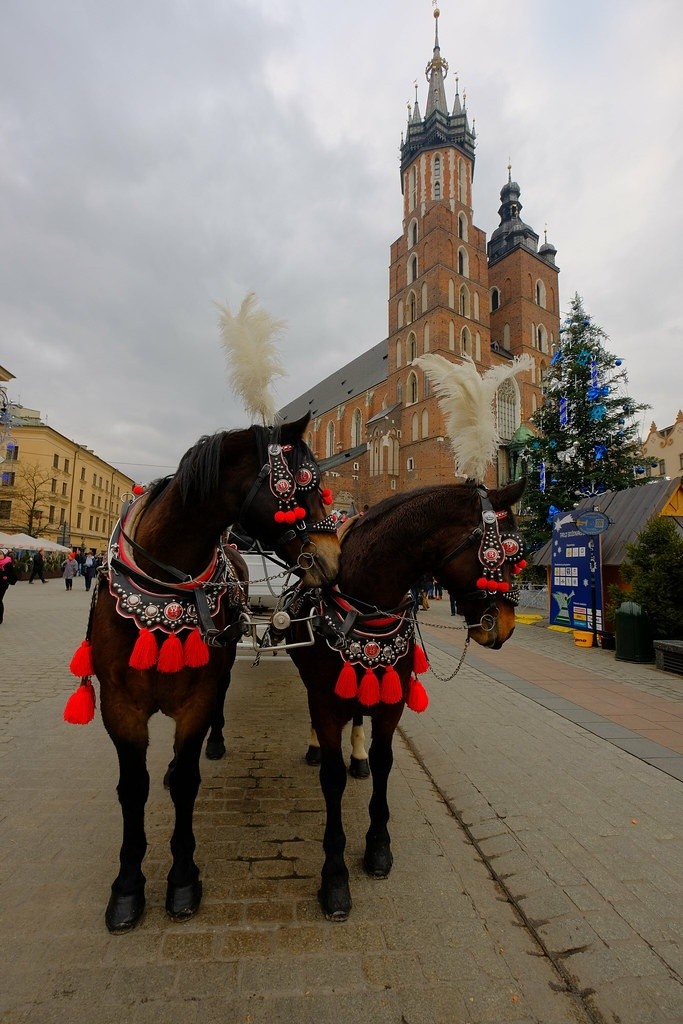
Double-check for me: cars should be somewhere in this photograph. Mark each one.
[412,582,442,598]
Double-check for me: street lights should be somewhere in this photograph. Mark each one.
[61,520,67,566]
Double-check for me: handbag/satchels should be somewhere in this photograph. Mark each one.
[73,568,77,577]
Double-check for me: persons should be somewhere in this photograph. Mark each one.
[29,549,49,584]
[76,550,82,576]
[61,552,78,591]
[416,579,462,617]
[0,548,16,624]
[83,552,104,591]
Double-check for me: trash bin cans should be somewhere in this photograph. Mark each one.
[614,602,657,664]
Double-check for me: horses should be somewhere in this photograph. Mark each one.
[90,407,341,936]
[285,458,529,924]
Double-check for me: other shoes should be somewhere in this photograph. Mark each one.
[451,613,456,616]
[86,588,89,591]
[428,596,435,600]
[66,589,69,591]
[70,587,72,590]
[420,607,430,611]
[435,597,442,600]
[42,581,49,584]
[29,582,35,584]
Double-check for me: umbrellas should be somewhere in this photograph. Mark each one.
[0,532,71,560]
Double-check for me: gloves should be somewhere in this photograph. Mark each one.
[63,563,67,567]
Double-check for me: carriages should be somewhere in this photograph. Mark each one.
[89,411,528,935]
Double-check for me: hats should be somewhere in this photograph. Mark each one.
[69,553,75,559]
[0,549,9,557]
